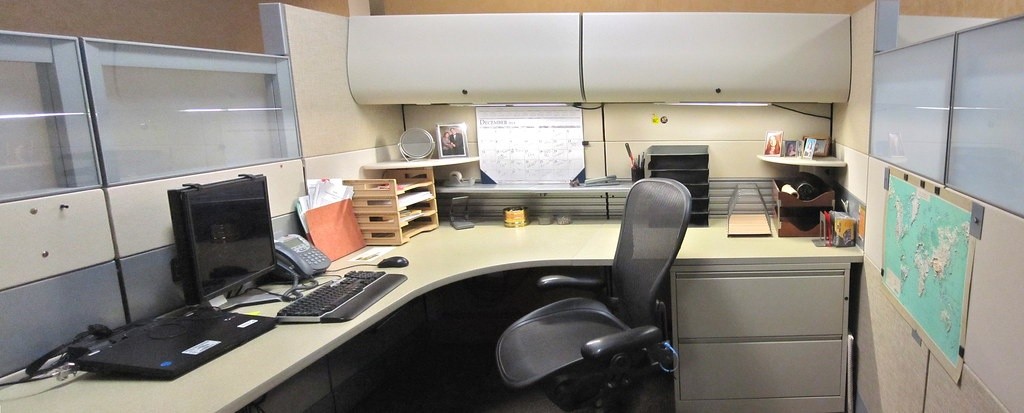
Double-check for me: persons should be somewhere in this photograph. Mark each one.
[766,134,781,155]
[804,141,813,158]
[814,143,821,153]
[797,144,802,156]
[785,143,796,156]
[442,128,464,154]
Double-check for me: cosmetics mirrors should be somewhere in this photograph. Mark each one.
[400,127,433,158]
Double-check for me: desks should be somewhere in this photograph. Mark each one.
[1,214,864,413]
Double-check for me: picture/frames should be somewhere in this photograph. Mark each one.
[435,123,468,159]
[763,130,831,161]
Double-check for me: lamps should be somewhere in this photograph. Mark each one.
[451,103,569,107]
[653,103,773,106]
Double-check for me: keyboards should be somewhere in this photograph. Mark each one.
[276,271,408,323]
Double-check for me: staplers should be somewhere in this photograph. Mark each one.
[584,175,620,187]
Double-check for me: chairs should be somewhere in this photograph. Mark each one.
[496,177,694,413]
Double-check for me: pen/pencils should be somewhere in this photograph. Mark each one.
[625,143,645,169]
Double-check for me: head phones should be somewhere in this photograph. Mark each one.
[25,324,113,377]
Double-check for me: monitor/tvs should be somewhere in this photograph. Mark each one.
[183,175,283,311]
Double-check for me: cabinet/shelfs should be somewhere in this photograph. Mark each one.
[343,168,439,246]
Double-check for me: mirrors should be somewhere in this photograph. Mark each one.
[397,127,436,162]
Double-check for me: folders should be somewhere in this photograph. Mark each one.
[304,198,368,263]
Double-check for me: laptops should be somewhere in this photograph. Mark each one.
[75,307,277,381]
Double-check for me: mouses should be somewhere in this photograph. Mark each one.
[377,256,409,268]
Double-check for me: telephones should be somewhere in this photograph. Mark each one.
[270,233,332,280]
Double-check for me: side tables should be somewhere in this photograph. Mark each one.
[669,262,853,413]
